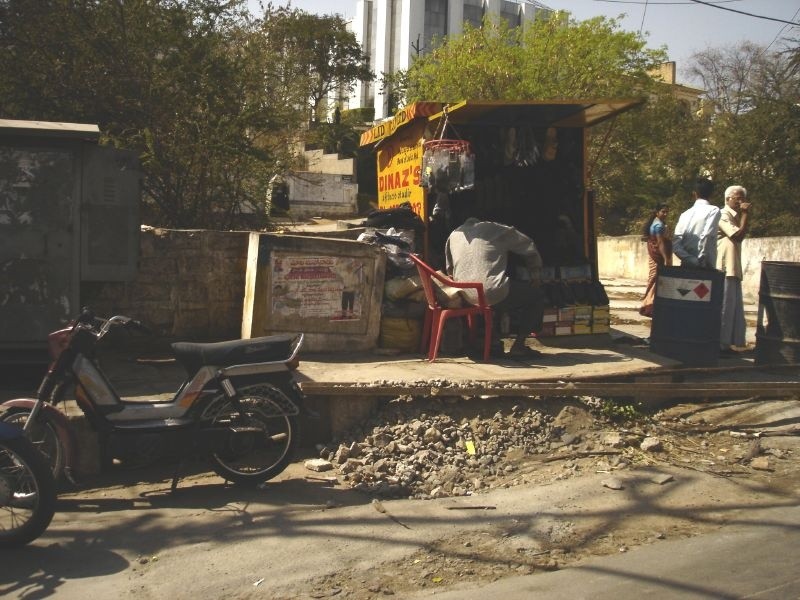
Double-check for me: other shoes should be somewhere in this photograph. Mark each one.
[719,347,742,358]
[503,347,540,364]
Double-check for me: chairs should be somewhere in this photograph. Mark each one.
[409,253,494,361]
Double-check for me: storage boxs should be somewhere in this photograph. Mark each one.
[530,304,611,337]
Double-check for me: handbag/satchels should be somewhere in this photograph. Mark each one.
[647,225,672,260]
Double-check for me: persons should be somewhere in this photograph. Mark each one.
[445,197,545,360]
[715,185,753,355]
[672,177,722,270]
[336,263,360,317]
[640,202,672,317]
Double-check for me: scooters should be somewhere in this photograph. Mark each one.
[0,304,322,512]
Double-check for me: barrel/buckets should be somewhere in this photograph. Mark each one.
[753,261,800,375]
[649,264,725,364]
